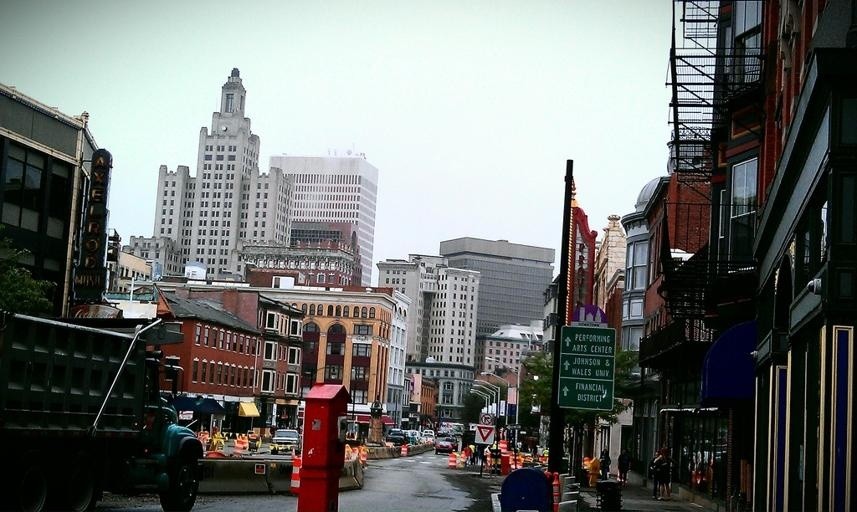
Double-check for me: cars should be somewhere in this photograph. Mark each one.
[434,438,459,454]
[387,428,449,444]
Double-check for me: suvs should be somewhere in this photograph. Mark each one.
[270,429,301,455]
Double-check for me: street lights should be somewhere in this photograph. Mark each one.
[485,356,522,448]
[470,372,511,427]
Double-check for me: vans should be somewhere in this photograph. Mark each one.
[346,420,366,445]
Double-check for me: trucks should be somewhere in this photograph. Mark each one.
[1,313,204,512]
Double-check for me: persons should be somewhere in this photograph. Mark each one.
[599,448,610,480]
[649,450,667,499]
[654,447,676,500]
[617,449,629,486]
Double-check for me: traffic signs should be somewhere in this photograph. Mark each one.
[558,378,613,411]
[505,424,522,430]
[561,325,615,357]
[560,353,614,381]
